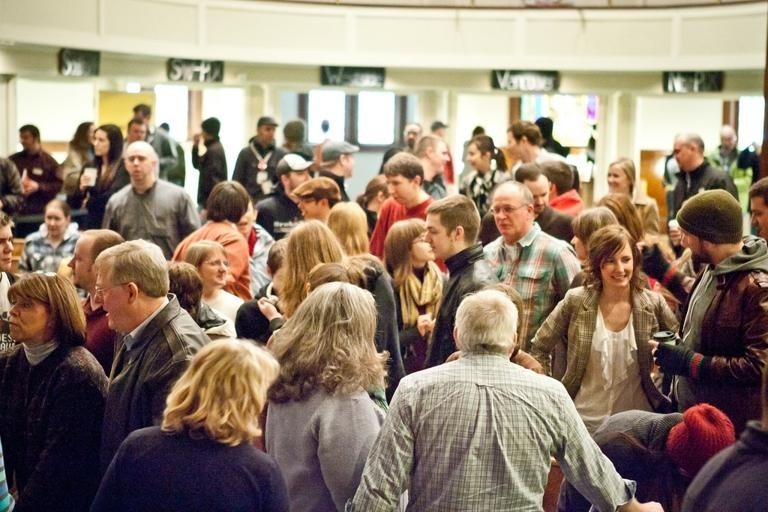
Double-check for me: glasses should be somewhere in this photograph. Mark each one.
[88,280,131,305]
[489,203,529,215]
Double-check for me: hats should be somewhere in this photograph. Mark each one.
[321,139,360,161]
[675,188,743,244]
[666,402,736,478]
[275,153,315,180]
[201,118,220,138]
[257,117,279,127]
[290,177,342,202]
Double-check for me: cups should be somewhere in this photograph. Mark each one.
[653,330,675,347]
[84,168,98,187]
[668,220,680,246]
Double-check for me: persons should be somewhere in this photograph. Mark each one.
[0,102,767,511]
